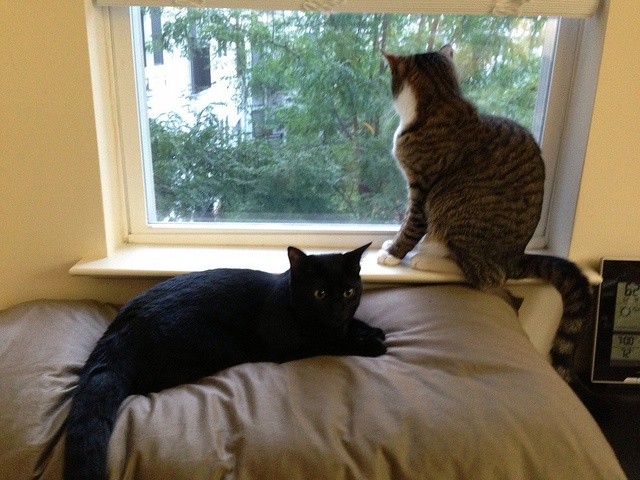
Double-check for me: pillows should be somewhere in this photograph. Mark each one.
[0,284,628,480]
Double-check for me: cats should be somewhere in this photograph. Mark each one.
[61,240,387,480]
[377,43,595,385]
